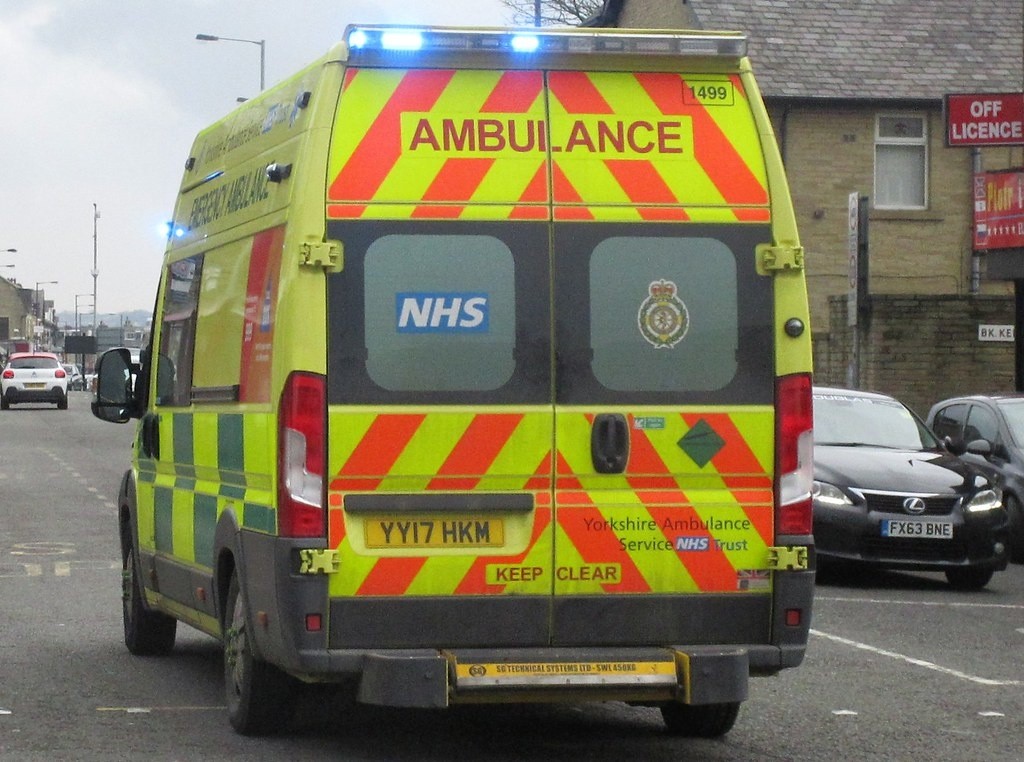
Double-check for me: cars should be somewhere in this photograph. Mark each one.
[809,384,1009,591]
[60,362,83,391]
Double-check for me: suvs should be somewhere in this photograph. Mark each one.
[926,395,1024,560]
[0,353,70,411]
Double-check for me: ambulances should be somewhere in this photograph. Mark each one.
[92,18,815,740]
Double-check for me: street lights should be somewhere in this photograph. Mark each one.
[75,293,95,364]
[93,313,117,371]
[35,281,59,350]
[194,33,266,93]
